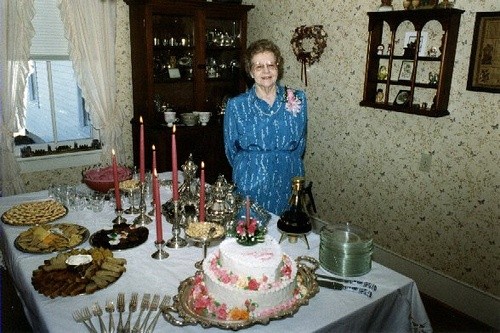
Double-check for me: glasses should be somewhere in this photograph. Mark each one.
[250,61,280,71]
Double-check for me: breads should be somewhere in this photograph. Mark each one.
[18,223,84,252]
[31,246,127,298]
[3,200,65,224]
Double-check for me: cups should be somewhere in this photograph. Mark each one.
[164,110,212,128]
[153,27,240,48]
[47,169,152,212]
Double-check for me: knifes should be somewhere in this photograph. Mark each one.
[317,280,372,298]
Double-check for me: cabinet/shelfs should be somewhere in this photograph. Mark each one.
[360,6,466,118]
[124,0,256,188]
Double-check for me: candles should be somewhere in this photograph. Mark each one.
[245,196,250,228]
[112,148,122,210]
[152,144,163,241]
[171,125,179,200]
[139,115,145,183]
[200,161,205,222]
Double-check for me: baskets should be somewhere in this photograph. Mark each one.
[81,162,133,194]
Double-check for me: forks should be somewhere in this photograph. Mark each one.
[72,293,172,333]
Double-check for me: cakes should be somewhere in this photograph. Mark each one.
[201,219,297,317]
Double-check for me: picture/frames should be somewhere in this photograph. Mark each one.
[467,11,500,94]
[398,61,414,81]
[404,31,427,56]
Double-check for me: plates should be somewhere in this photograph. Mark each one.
[319,223,373,278]
[14,224,89,254]
[1,205,69,226]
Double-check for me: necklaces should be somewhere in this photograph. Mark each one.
[223,38,307,218]
[255,96,282,116]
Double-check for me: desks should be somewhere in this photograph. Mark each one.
[0,169,434,333]
